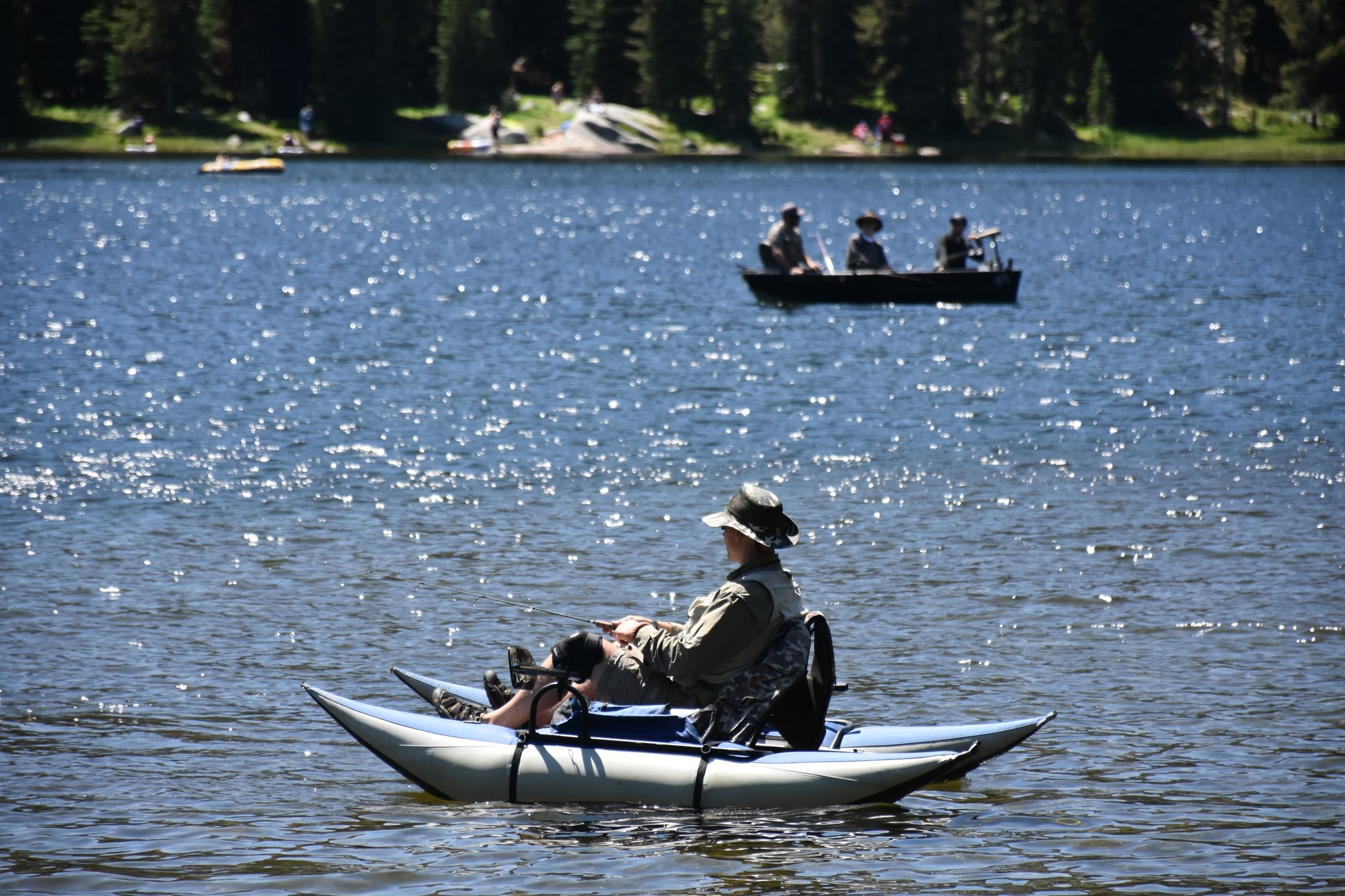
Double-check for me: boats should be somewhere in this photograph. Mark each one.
[446,140,492,154]
[301,597,1057,804]
[734,226,1024,304]
[198,158,286,174]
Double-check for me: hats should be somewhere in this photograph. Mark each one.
[856,210,882,232]
[782,202,806,217]
[702,484,802,551]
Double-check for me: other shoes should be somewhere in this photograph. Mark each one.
[432,687,486,723]
[482,670,517,710]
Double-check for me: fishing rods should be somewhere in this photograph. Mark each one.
[278,552,619,632]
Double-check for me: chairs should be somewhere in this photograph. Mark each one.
[729,608,851,752]
[758,243,788,275]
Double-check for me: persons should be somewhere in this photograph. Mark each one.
[846,210,897,274]
[877,112,898,154]
[431,483,811,729]
[301,105,317,138]
[767,204,824,274]
[490,106,503,150]
[934,213,985,271]
